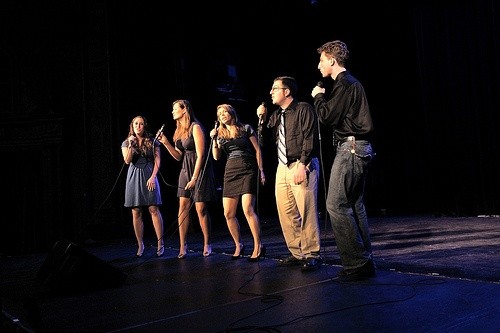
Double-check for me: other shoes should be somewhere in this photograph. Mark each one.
[345,262,370,280]
[334,257,343,264]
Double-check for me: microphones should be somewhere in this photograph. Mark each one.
[153,124,166,142]
[258,102,265,125]
[129,131,135,147]
[318,80,324,88]
[212,121,220,139]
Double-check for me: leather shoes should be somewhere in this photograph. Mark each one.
[284,256,304,264]
[302,257,321,270]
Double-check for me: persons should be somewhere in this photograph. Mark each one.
[257,77,321,272]
[210,104,267,262]
[121,116,165,259]
[157,100,212,258]
[310,40,376,279]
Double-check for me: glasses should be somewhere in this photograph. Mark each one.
[272,87,287,91]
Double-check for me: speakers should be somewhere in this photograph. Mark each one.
[26,239,123,291]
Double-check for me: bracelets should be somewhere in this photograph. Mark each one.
[192,177,197,180]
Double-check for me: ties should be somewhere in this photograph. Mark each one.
[278,110,287,165]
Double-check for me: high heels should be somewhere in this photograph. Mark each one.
[232,244,244,260]
[177,245,187,258]
[137,245,144,257]
[203,245,211,256]
[157,245,164,256]
[248,245,266,262]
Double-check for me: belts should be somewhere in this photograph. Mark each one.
[287,154,317,161]
[340,135,369,145]
